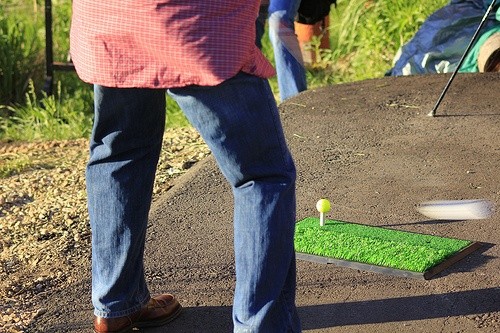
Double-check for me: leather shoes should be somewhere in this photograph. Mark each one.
[93,293,183,333]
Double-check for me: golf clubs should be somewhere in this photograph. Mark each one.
[315,199,331,212]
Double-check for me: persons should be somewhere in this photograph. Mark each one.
[259,1,308,107]
[292,1,339,83]
[67,1,302,333]
